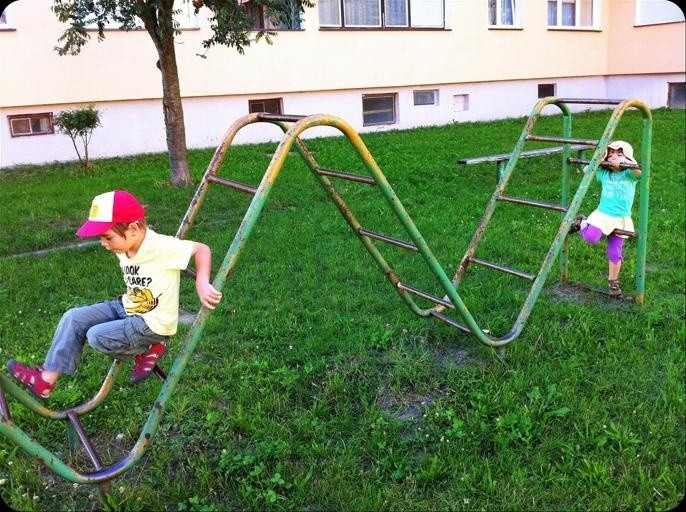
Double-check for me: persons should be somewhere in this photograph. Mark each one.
[7,189,222,401]
[568,140,641,296]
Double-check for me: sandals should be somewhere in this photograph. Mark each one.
[129,342,167,384]
[6,358,56,401]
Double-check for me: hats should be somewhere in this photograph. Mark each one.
[76,189,145,238]
[603,139,637,163]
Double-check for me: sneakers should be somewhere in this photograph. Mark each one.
[569,214,587,234]
[608,278,621,297]
